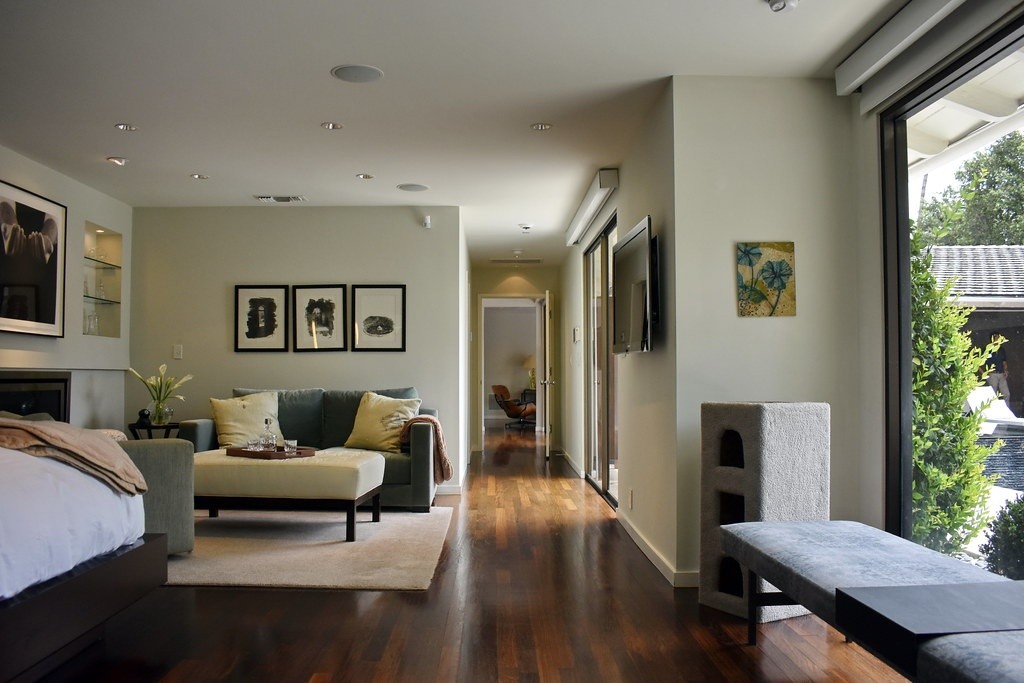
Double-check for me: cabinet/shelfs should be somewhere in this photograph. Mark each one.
[83,220,122,339]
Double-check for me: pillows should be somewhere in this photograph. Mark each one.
[343,391,423,454]
[209,391,284,450]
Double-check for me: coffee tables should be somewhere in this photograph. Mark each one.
[192,448,386,542]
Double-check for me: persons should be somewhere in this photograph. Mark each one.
[976,332,1011,407]
[0,199,58,264]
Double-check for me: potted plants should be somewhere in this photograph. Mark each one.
[129,363,194,426]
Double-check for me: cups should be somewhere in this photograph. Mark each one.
[283,440,297,456]
[247,440,259,451]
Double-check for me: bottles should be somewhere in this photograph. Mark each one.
[87,311,99,335]
[260,418,277,452]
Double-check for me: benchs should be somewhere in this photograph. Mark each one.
[718,521,1023,682]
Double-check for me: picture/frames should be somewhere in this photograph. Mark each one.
[352,283,406,352]
[1,180,68,338]
[234,284,288,352]
[293,283,348,351]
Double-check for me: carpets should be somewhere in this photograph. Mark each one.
[162,506,454,591]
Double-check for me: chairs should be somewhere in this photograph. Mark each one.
[491,384,536,430]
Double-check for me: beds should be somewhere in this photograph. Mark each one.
[0,434,169,683]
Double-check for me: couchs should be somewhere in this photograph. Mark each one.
[178,388,439,514]
[19,412,195,558]
